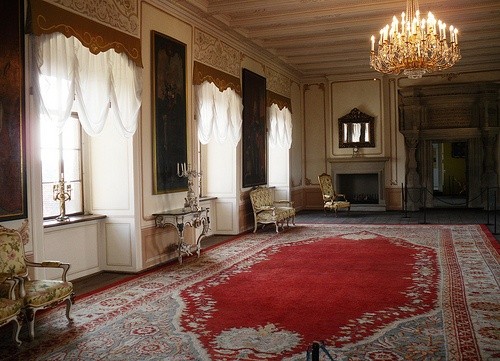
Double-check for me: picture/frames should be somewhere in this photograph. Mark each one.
[240,65,267,189]
[150,28,189,195]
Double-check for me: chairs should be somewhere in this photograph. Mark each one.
[0,277,24,347]
[0,225,75,342]
[317,172,352,218]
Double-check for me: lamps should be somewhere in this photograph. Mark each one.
[369,0,462,81]
[52,172,72,222]
[176,160,204,211]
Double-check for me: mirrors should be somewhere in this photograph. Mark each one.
[338,107,376,149]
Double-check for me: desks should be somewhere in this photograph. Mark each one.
[151,206,210,266]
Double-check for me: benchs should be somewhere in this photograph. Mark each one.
[249,184,296,234]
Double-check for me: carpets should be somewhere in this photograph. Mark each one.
[0,221,500,361]
